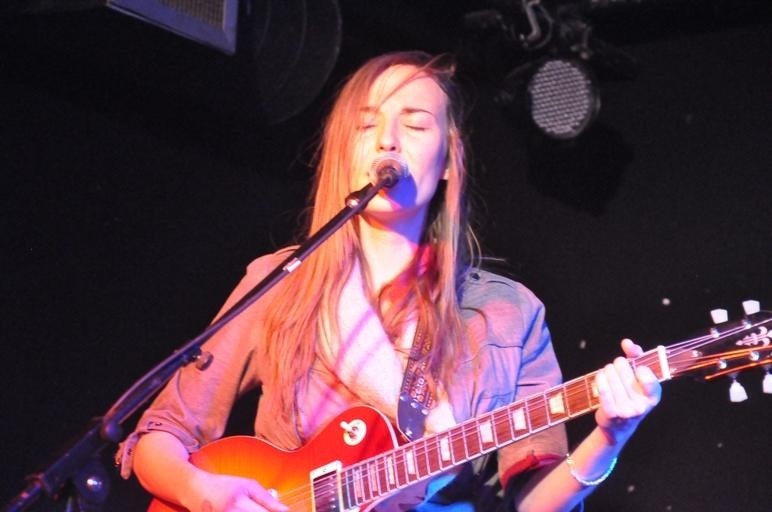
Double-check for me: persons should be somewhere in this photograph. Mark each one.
[116,50,662,512]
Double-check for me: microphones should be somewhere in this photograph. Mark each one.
[368,150,410,190]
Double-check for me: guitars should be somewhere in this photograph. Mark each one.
[147,299,771,511]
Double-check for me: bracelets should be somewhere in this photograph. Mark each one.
[565,453,618,486]
